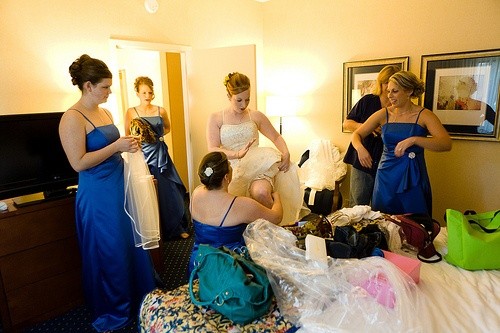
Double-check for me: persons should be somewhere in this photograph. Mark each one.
[190,72,304,279]
[351,71,452,230]
[59,54,160,332]
[342,65,401,205]
[125,76,190,240]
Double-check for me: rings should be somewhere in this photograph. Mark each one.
[399,148,402,151]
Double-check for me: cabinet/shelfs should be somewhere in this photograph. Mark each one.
[0,179,166,333]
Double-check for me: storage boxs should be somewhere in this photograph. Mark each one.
[364,249,421,309]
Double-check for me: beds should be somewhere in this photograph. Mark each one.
[141,220,499,333]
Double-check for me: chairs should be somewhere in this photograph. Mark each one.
[300,146,350,216]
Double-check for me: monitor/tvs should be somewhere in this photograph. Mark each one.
[0,112,80,209]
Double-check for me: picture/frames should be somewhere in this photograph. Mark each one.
[341,56,409,132]
[418,49,500,142]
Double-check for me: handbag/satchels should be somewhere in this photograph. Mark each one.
[390,213,440,258]
[189,244,280,326]
[443,208,500,271]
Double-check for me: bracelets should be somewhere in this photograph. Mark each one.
[238,152,241,162]
